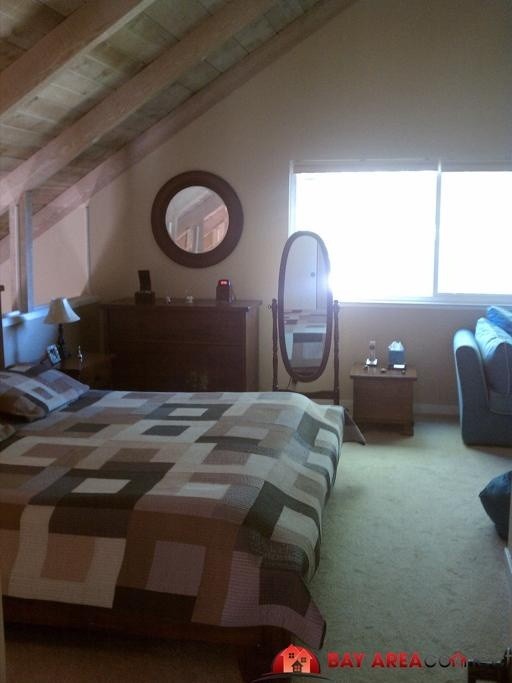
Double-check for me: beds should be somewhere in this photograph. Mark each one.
[284,309,328,373]
[0,389,346,683]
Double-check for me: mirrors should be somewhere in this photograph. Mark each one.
[150,170,243,269]
[267,230,340,407]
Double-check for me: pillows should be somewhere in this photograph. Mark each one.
[0,363,91,452]
[474,308,511,394]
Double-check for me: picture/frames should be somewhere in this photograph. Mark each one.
[40,344,61,366]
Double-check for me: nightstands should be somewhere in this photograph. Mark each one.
[55,352,118,391]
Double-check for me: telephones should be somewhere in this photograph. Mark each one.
[366,338,379,366]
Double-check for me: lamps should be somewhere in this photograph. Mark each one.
[43,297,82,360]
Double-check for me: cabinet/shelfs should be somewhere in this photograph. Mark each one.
[349,365,417,437]
[98,297,261,393]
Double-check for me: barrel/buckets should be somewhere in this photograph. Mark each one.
[387,336,405,369]
